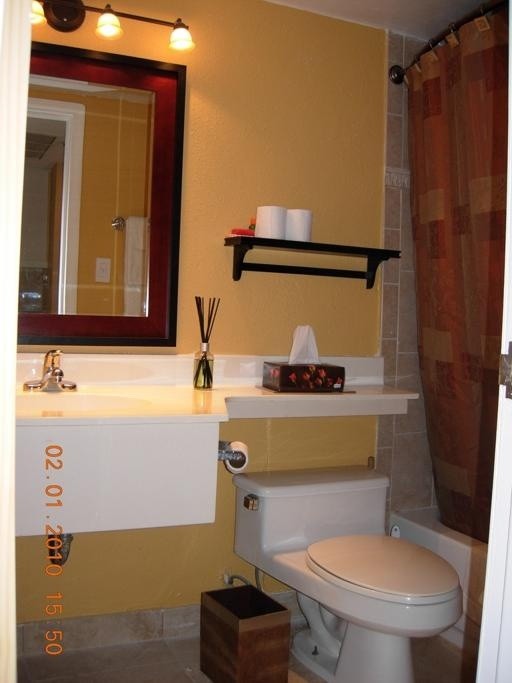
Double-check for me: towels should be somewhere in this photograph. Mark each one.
[124,216,145,315]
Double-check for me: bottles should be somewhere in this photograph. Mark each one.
[194,342,214,391]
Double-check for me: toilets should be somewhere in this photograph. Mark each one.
[233,465,464,683]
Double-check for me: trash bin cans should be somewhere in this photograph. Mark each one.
[199,584,291,683]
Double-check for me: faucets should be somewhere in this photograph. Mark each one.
[22,350,79,391]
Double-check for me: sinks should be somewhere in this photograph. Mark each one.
[15,395,133,418]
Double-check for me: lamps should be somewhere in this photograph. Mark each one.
[31,0,196,52]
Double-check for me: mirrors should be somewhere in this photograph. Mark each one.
[17,41,187,347]
